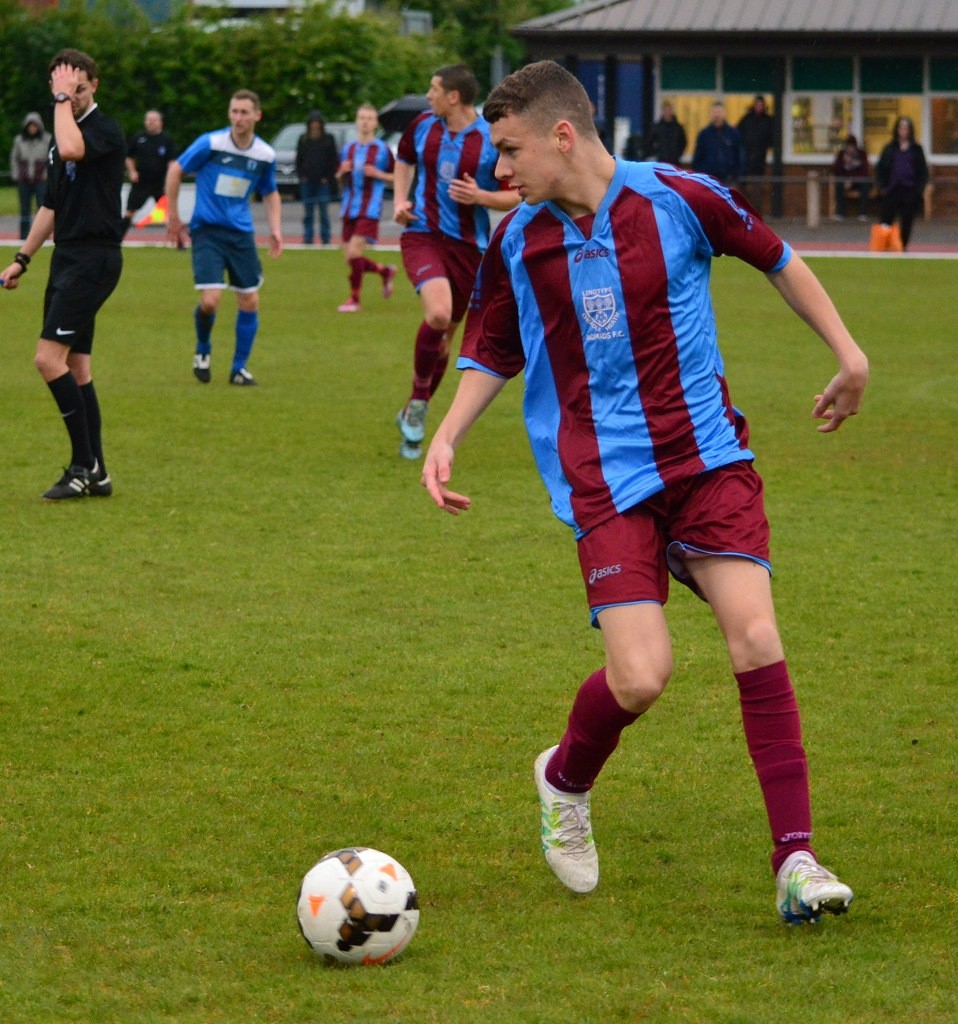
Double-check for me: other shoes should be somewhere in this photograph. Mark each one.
[834,215,868,222]
[175,240,185,252]
[902,246,909,253]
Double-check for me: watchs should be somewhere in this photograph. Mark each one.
[53,92,70,103]
[14,252,30,272]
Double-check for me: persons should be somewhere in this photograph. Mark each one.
[118,64,522,461]
[627,96,931,252]
[0,48,127,503]
[9,112,53,240]
[420,61,868,929]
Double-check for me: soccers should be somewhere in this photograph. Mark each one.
[295,843,420,967]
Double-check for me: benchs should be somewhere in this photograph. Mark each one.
[830,171,934,223]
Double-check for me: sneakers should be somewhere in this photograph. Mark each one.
[397,400,428,459]
[43,457,112,499]
[338,300,360,312]
[534,744,599,892]
[193,354,210,383]
[229,369,256,385]
[777,851,853,925]
[383,265,398,298]
